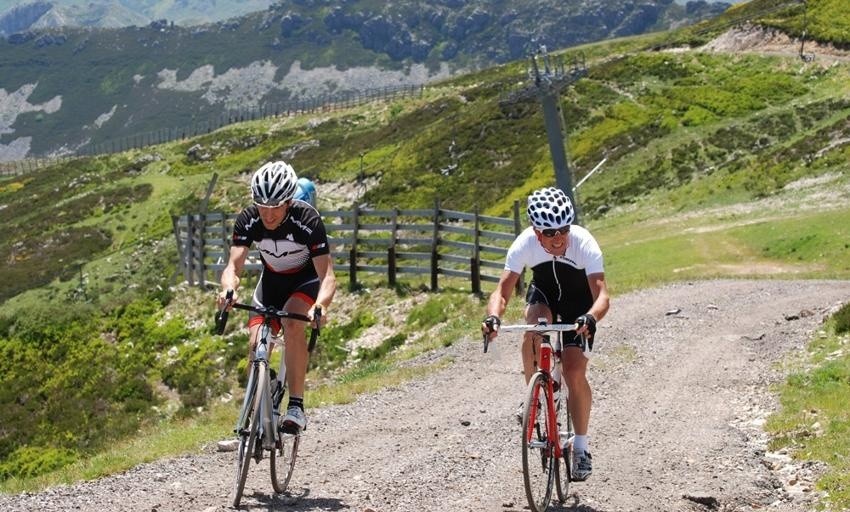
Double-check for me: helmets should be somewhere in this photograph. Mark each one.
[527,187,576,229]
[251,161,299,208]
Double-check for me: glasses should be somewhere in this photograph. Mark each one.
[542,227,570,235]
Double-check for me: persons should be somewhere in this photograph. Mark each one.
[213,157,338,436]
[478,185,611,484]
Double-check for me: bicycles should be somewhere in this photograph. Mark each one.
[482,316,586,511]
[214,288,322,507]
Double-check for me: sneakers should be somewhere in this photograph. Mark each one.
[281,407,308,431]
[571,452,592,481]
[514,399,541,423]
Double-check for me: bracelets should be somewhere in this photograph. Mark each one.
[314,303,328,311]
